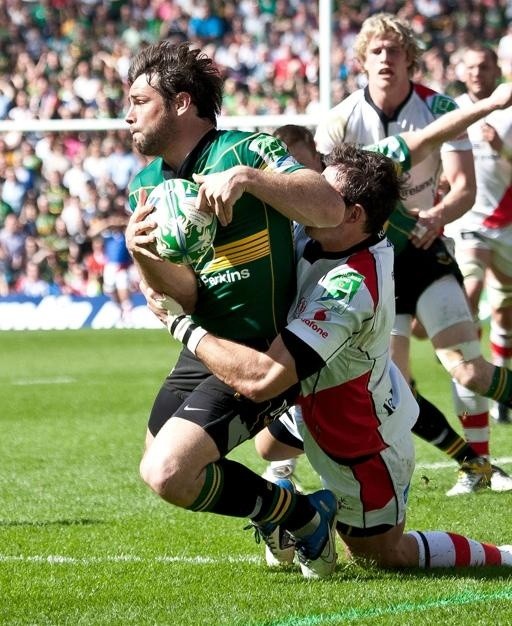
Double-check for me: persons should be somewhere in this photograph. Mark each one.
[1,1,320,120]
[125,38,346,580]
[0,129,142,309]
[139,144,512,571]
[254,11,512,494]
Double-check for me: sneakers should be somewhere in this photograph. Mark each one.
[256,461,339,579]
[445,457,512,496]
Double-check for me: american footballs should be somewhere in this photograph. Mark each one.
[145,179,217,265]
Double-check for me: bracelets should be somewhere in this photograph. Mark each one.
[168,316,203,355]
